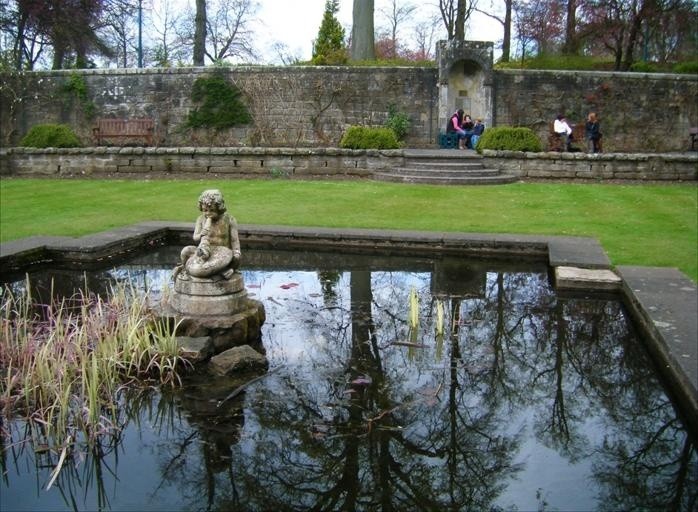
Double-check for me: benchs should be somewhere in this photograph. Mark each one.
[92,119,157,147]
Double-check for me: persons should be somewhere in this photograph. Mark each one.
[461,115,473,149]
[586,112,602,153]
[470,119,484,149]
[553,113,572,152]
[446,109,468,150]
[176,188,241,281]
